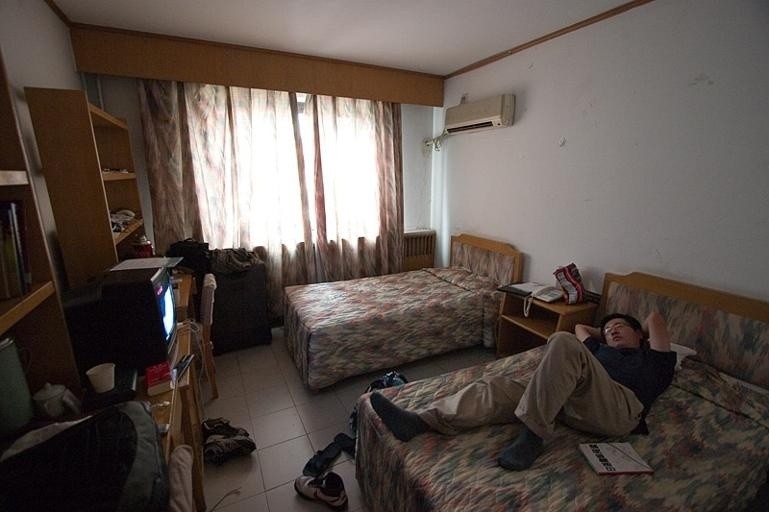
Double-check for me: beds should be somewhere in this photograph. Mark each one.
[283,232,524,396]
[354,271,769,511]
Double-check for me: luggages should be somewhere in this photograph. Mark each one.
[195,248,272,357]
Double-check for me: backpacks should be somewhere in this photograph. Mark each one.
[348,370,409,433]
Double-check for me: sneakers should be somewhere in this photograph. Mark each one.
[203,434,256,466]
[294,471,348,511]
[202,416,249,440]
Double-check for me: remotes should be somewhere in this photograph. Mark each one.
[175,354,195,379]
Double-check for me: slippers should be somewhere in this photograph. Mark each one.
[302,442,342,478]
[334,433,356,458]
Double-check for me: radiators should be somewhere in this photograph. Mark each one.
[404,230,436,272]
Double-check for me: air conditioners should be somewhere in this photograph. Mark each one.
[444,93,517,135]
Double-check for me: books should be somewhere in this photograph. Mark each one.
[146,360,176,396]
[0,199,34,302]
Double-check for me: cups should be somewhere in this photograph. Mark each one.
[84,363,116,394]
[32,382,68,419]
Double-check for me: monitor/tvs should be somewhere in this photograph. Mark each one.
[63,267,177,361]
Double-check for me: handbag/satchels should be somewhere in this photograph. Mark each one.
[167,237,211,272]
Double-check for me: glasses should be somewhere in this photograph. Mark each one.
[603,323,631,336]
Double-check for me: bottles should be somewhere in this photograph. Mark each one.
[129,233,155,258]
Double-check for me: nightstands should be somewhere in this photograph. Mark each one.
[493,279,598,361]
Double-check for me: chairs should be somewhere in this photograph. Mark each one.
[189,274,221,404]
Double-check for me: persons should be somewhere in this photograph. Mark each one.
[369,310,676,472]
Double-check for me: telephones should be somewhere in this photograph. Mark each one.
[116,210,135,219]
[532,285,564,302]
